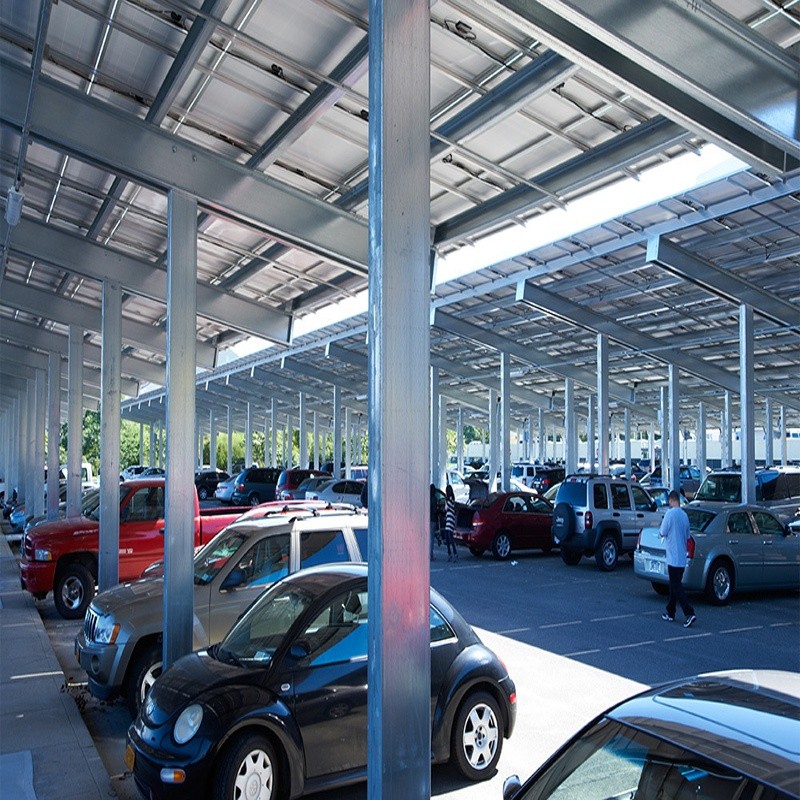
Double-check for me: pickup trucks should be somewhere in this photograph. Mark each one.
[19,478,287,619]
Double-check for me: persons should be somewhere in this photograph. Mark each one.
[657,490,696,627]
[430,484,439,561]
[361,476,368,508]
[443,485,458,562]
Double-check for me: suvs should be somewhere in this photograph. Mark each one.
[552,473,668,571]
[74,503,370,719]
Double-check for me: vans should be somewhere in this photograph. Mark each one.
[690,464,799,520]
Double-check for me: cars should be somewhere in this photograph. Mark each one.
[440,491,553,562]
[644,487,689,507]
[276,470,331,501]
[214,473,243,506]
[0,463,165,559]
[232,468,282,506]
[195,472,231,501]
[650,464,715,500]
[503,669,799,800]
[128,562,517,800]
[634,503,800,607]
[280,477,334,500]
[292,462,369,482]
[437,455,652,510]
[305,480,364,509]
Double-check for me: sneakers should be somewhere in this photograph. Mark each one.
[661,614,674,622]
[684,615,696,628]
